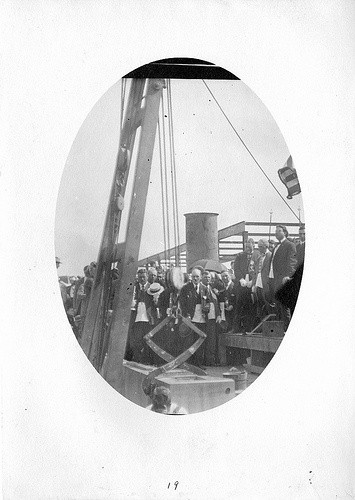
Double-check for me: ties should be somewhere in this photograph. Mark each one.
[268,242,281,272]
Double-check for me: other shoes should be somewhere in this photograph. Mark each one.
[216,361,227,367]
[228,328,242,334]
[206,360,216,366]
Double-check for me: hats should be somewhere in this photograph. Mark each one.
[146,282,164,296]
[56,257,62,264]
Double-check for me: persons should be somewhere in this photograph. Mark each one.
[144,385,187,415]
[54,225,306,371]
